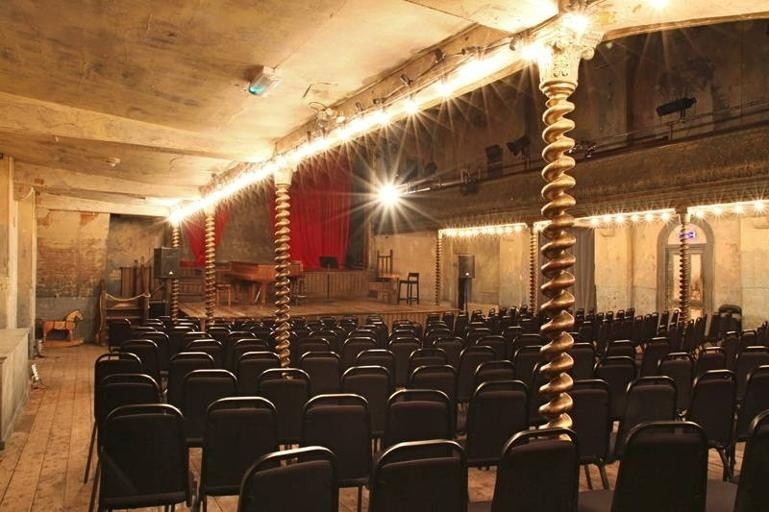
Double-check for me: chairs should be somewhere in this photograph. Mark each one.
[398,272,420,305]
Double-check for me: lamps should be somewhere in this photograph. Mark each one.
[506,135,530,156]
[657,98,696,122]
